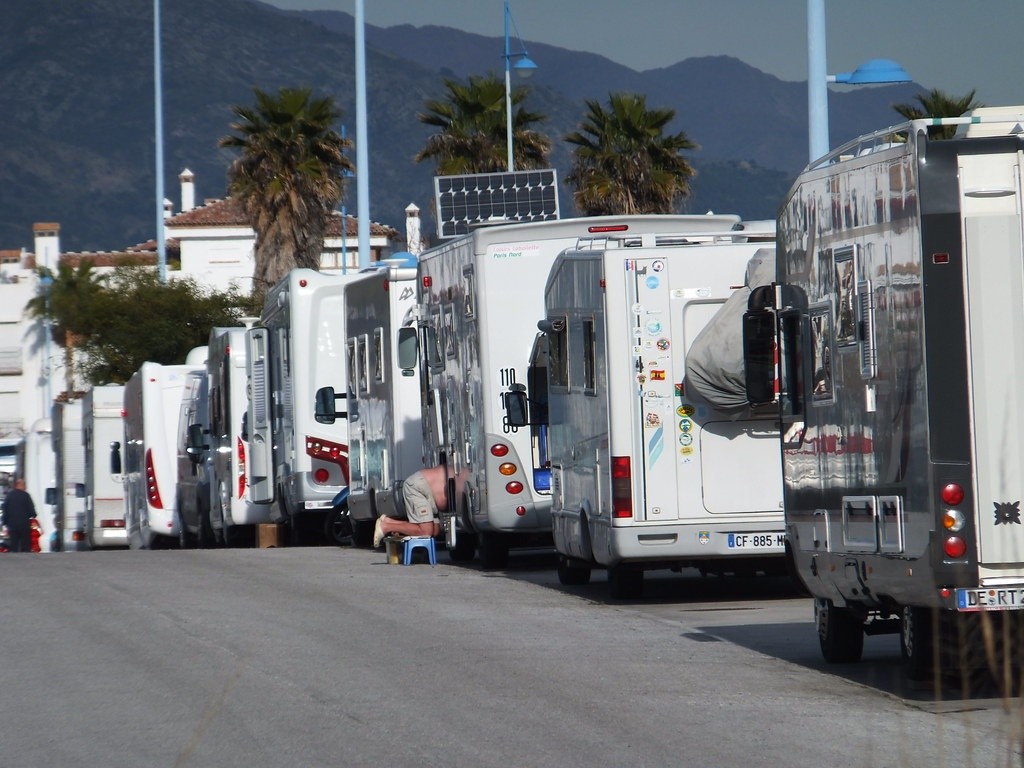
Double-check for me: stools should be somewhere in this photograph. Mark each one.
[384,537,402,565]
[403,536,437,566]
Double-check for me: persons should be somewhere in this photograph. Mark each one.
[2,479,37,553]
[374,464,448,548]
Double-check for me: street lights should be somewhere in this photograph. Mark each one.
[39,273,55,371]
[499,1,537,177]
[337,124,354,277]
[809,0,913,171]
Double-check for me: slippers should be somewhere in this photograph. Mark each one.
[373,514,387,547]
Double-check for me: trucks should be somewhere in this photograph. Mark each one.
[396,214,742,572]
[312,266,424,544]
[742,114,1020,682]
[503,230,789,604]
[0,267,376,551]
